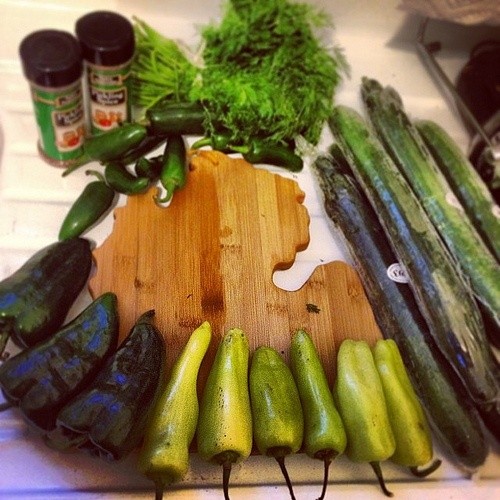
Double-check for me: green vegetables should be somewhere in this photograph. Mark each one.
[129,0,345,148]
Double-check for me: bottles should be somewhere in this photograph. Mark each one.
[75,10,140,136]
[19,30,92,171]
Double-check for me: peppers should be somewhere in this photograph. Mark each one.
[0,238,166,463]
[140,321,443,500]
[58,104,304,239]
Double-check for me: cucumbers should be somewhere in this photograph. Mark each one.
[313,75,500,469]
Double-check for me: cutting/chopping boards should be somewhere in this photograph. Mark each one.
[87,150,388,456]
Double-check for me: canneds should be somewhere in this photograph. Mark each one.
[76,10,136,135]
[19,30,88,167]
